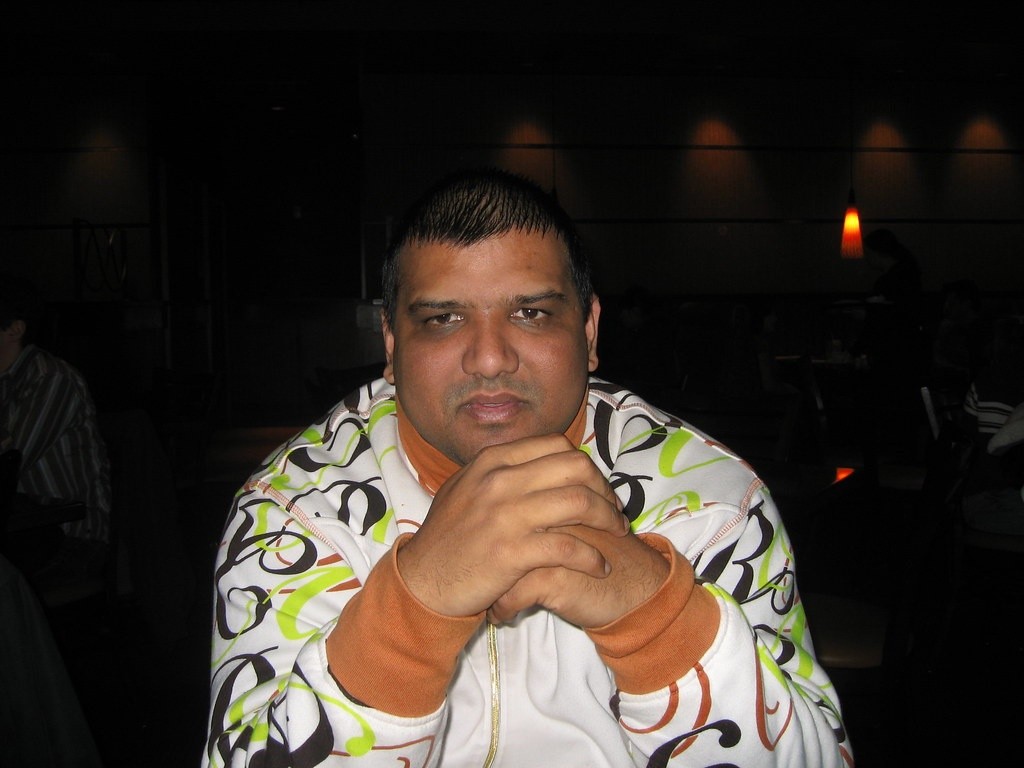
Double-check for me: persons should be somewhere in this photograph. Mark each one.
[840,227,1024,522]
[0,277,112,768]
[198,170,853,768]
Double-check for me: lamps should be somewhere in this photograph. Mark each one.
[840,78,866,260]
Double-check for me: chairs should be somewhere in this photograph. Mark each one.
[93,289,1024,719]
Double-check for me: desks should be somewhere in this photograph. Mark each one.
[965,492,1024,553]
[204,425,314,475]
